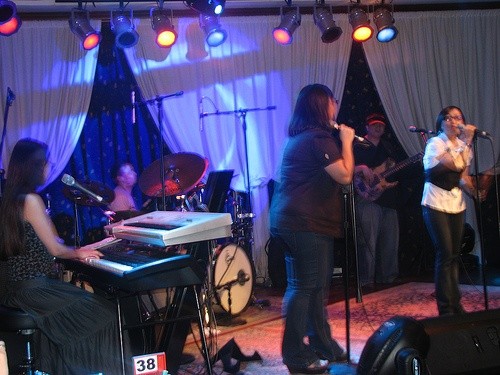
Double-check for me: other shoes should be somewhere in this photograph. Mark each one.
[286,358,329,375]
[178,354,195,365]
[336,351,348,360]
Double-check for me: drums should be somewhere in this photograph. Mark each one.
[201,242,253,316]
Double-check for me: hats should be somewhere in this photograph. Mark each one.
[365,115,386,127]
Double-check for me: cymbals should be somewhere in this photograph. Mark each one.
[139,151,208,198]
[63,180,116,207]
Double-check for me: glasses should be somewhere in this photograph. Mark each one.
[442,115,463,121]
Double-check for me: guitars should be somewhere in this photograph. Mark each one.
[354,148,425,201]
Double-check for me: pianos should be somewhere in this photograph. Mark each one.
[55,211,233,375]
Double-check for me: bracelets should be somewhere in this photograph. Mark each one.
[466,142,472,147]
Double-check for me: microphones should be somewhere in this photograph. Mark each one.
[408,125,435,134]
[132,88,136,124]
[330,120,367,143]
[239,272,249,281]
[61,173,103,202]
[458,124,490,136]
[199,101,203,132]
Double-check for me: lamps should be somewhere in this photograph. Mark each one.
[69,0,102,50]
[313,0,343,44]
[347,0,399,43]
[150,0,179,48]
[111,0,139,48]
[272,0,301,46]
[182,0,227,46]
[0,0,23,37]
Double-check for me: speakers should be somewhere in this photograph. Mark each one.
[355,307,500,375]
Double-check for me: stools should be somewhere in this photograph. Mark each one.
[0,310,49,375]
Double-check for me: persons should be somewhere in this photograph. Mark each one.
[0,141,133,375]
[353,113,403,290]
[420,106,487,318]
[268,84,355,374]
[108,161,138,212]
